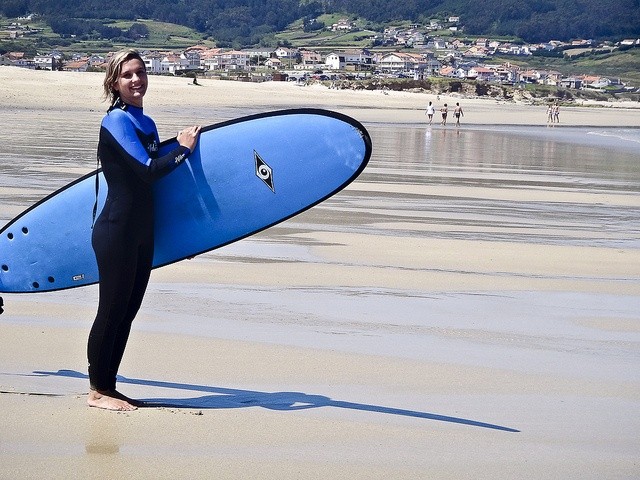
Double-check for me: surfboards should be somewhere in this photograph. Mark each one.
[0,107,372,293]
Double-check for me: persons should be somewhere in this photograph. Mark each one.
[439,103,451,126]
[452,102,464,127]
[87,48,203,413]
[546,102,560,124]
[425,99,436,126]
